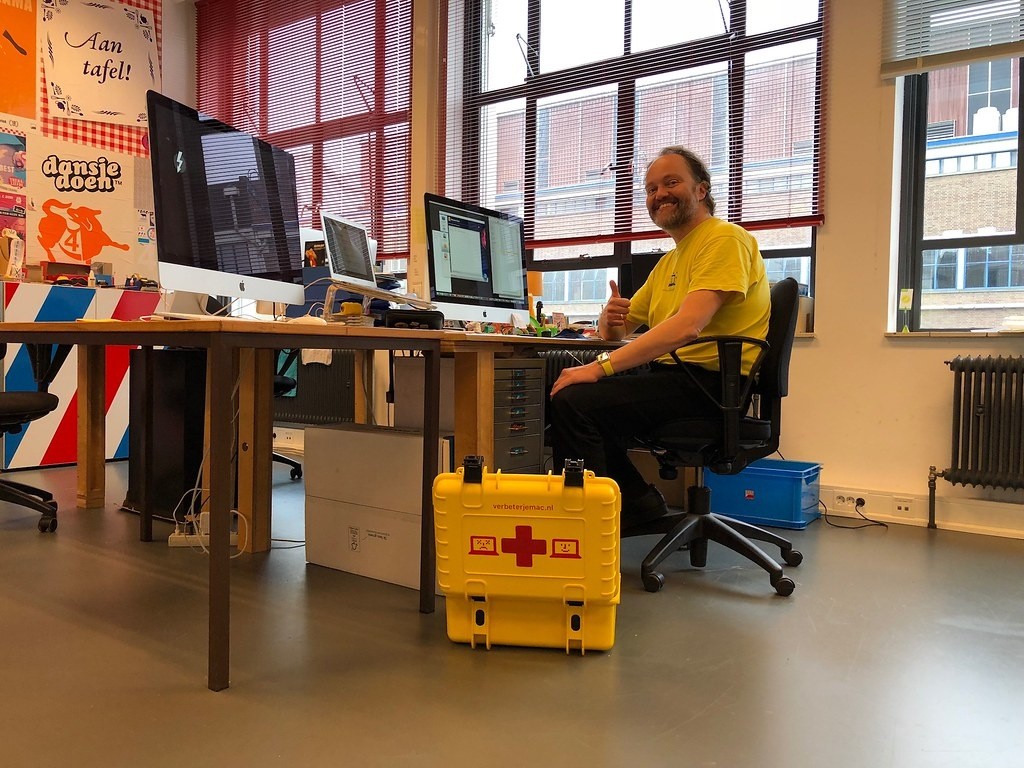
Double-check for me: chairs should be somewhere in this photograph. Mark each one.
[624,277,803,597]
[0,343,59,534]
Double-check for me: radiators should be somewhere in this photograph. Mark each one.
[273,349,355,425]
[944,356,1024,493]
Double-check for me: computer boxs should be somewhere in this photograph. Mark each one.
[301,424,454,598]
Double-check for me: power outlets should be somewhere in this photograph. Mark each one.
[833,489,870,513]
[283,432,295,444]
[892,495,915,518]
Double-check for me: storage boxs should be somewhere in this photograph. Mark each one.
[303,422,454,598]
[703,458,824,531]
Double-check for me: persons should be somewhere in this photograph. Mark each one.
[549,147,772,531]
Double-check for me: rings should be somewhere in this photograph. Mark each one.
[621,314,623,320]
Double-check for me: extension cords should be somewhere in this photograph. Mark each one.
[167,531,239,546]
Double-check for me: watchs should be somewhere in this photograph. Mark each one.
[596,352,614,376]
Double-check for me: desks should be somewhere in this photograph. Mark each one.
[0,321,446,692]
[440,332,689,512]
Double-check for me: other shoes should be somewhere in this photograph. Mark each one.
[621,484,669,529]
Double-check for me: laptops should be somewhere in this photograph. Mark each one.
[318,209,433,305]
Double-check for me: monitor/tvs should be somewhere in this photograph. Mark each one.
[145,89,307,322]
[423,192,531,331]
